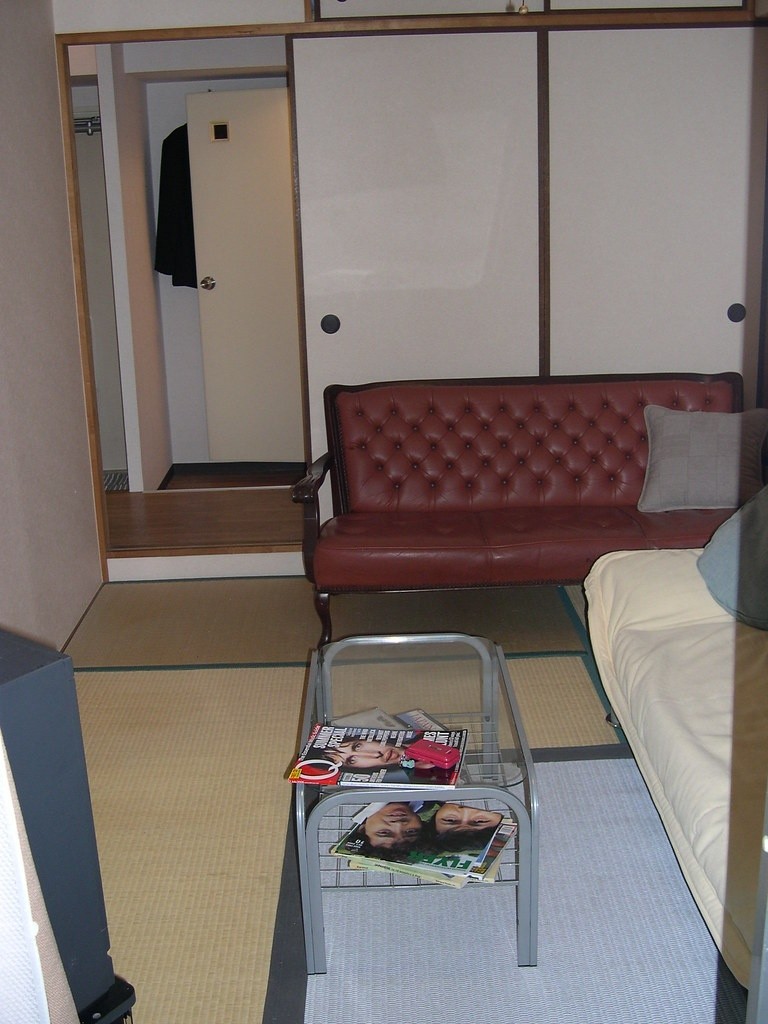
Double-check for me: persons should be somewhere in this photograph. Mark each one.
[421,802,503,853]
[360,801,435,862]
[303,732,459,785]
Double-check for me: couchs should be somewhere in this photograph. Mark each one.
[580,494,768,1024]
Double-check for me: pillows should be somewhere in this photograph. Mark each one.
[696,484,768,631]
[637,405,768,512]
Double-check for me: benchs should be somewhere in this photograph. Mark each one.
[290,371,764,648]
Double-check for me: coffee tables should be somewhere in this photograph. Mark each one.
[292,633,541,976]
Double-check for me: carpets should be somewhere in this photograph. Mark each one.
[261,744,748,1024]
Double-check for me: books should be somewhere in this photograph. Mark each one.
[329,801,518,891]
[287,724,469,789]
